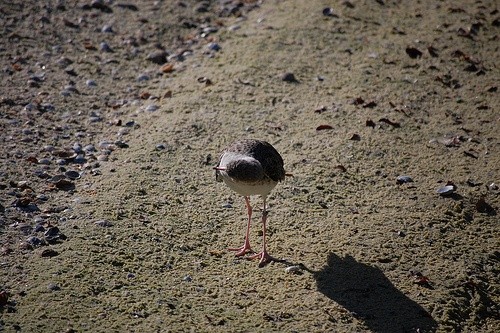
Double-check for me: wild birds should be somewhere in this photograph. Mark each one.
[212,138,285,268]
